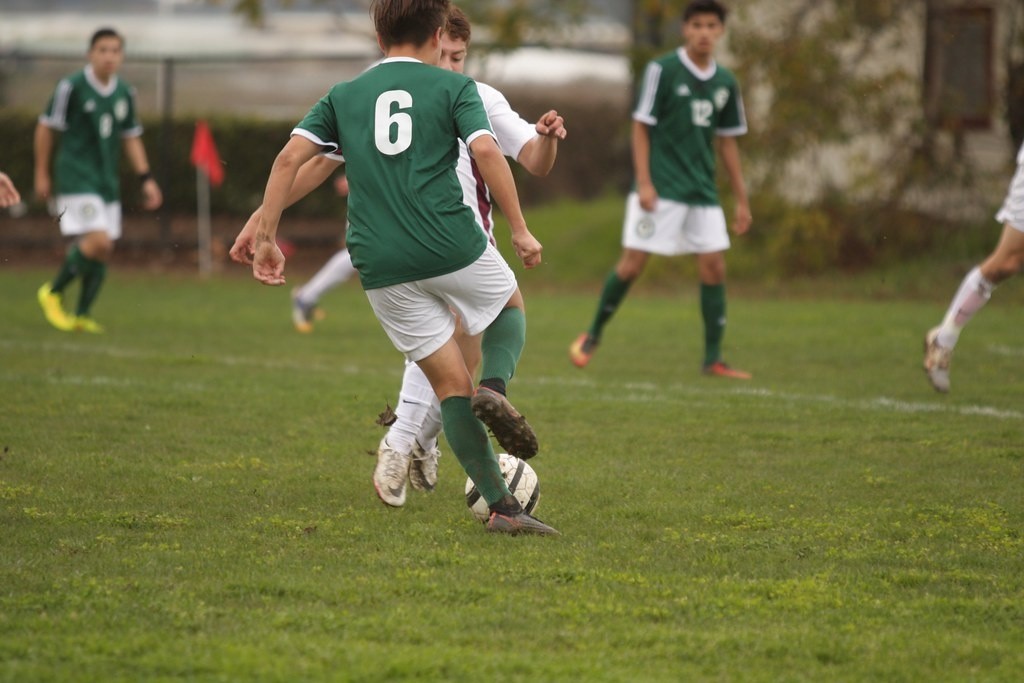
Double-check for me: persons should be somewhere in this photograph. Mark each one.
[0,28,164,335]
[568,0,756,380]
[922,138,1024,395]
[229,0,567,538]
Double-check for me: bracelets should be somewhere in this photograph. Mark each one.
[134,171,154,188]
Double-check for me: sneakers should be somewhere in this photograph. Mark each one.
[292,290,318,334]
[472,387,538,461]
[74,316,102,333]
[38,282,76,330]
[703,362,751,378]
[374,433,413,507]
[570,334,595,367]
[925,327,955,393]
[486,510,563,539]
[408,437,441,498]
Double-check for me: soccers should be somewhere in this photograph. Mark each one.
[465,452,543,523]
